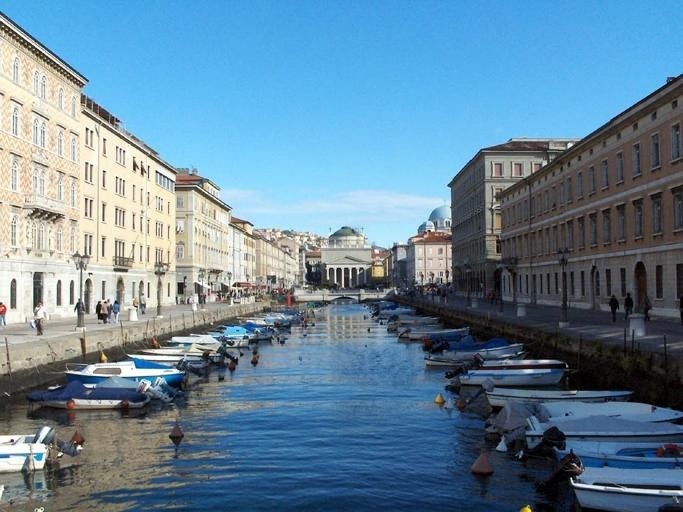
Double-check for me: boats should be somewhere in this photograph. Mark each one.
[0,426,85,473]
[26,310,294,411]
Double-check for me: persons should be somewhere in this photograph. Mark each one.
[397,282,452,301]
[0,302,7,325]
[73,298,85,321]
[216,289,266,302]
[34,301,48,335]
[623,293,633,320]
[640,292,652,322]
[95,292,148,324]
[679,292,683,325]
[489,288,501,307]
[609,295,620,322]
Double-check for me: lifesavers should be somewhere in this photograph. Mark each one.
[657,443,683,457]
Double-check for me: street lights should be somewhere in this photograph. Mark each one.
[465,266,472,309]
[420,274,424,297]
[73,250,90,327]
[227,271,232,298]
[154,261,165,316]
[445,270,449,303]
[557,248,570,322]
[430,273,435,304]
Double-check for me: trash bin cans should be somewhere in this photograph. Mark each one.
[516,304,526,317]
[128,307,138,321]
[227,297,234,305]
[627,313,646,337]
[192,302,198,311]
[471,298,479,308]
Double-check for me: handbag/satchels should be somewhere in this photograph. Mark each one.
[30,320,37,329]
